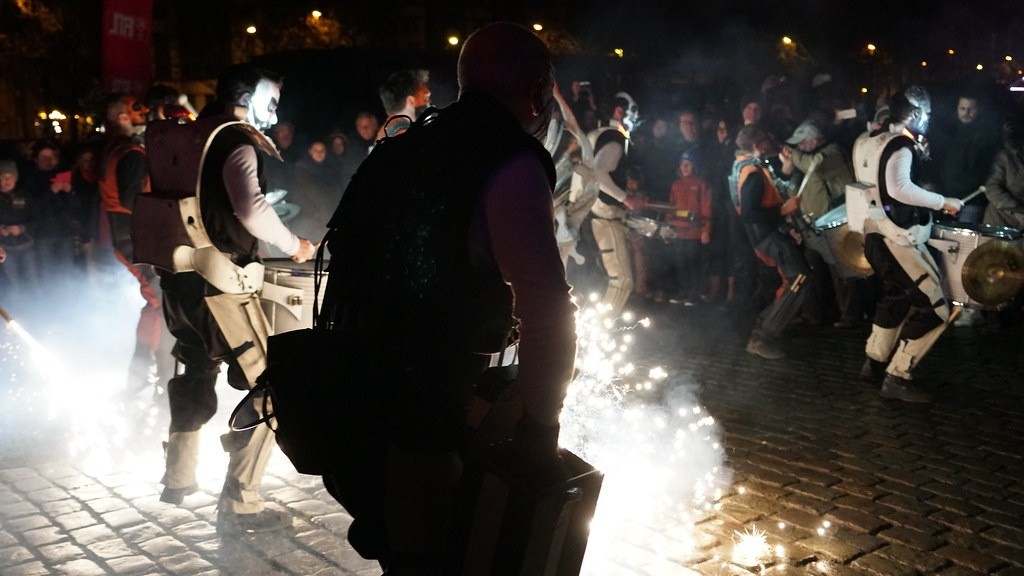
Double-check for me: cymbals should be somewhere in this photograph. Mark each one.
[832,222,874,274]
[960,239,1021,306]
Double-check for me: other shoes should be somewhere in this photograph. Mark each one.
[877,374,932,403]
[670,295,685,304]
[684,297,698,306]
[216,507,294,535]
[745,329,787,359]
[860,359,886,377]
[160,482,198,504]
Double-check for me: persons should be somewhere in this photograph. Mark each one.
[732,127,807,360]
[105,82,198,410]
[851,88,964,404]
[139,64,323,527]
[1,1,1024,402]
[324,22,577,575]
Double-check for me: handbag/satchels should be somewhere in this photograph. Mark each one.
[229,329,375,477]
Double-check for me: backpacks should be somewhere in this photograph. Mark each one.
[312,104,506,347]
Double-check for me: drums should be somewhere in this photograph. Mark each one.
[261,259,330,337]
[814,202,876,278]
[929,215,1022,314]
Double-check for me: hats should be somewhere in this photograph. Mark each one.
[681,144,702,169]
